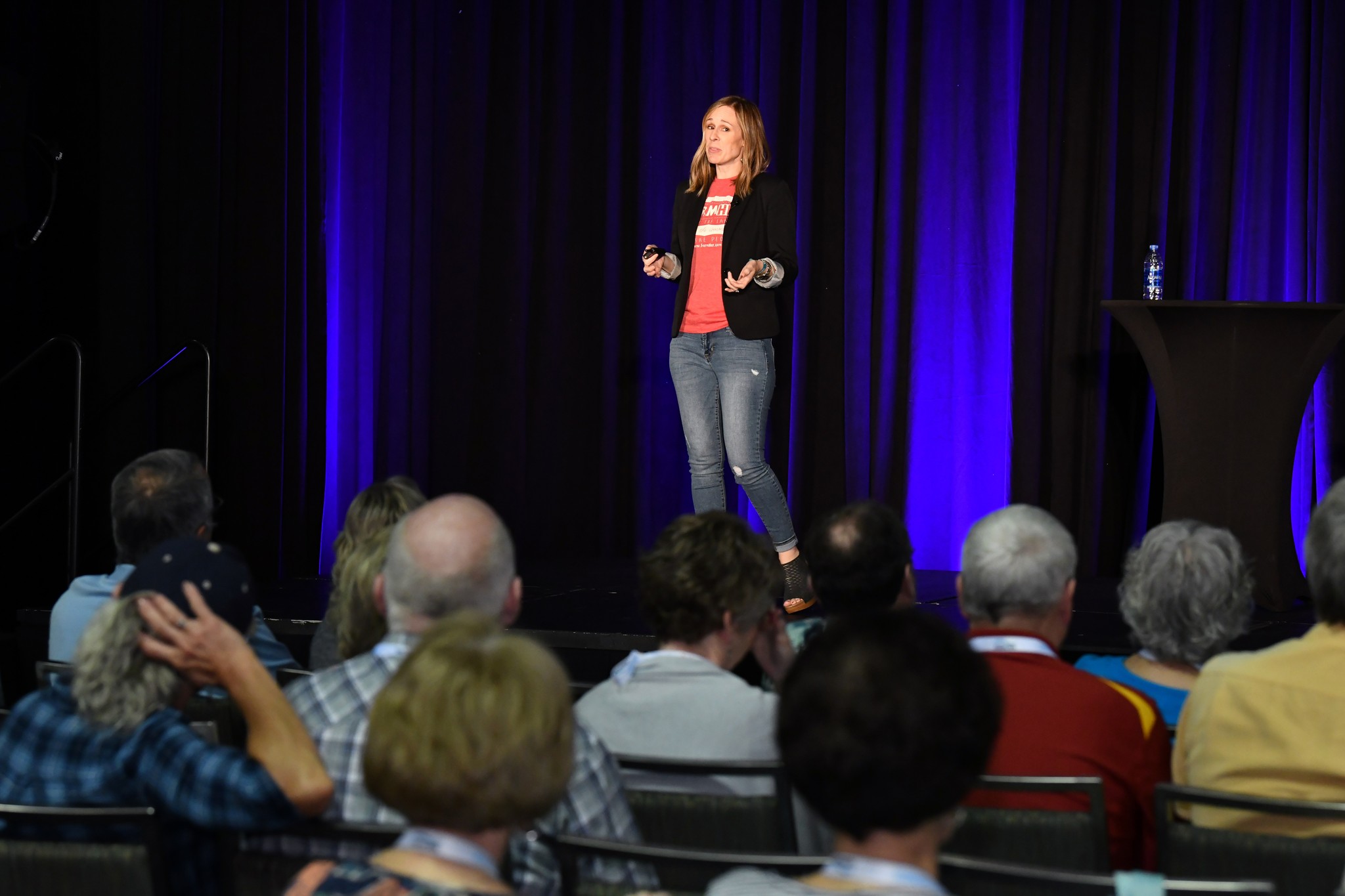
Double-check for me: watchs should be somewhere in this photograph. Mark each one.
[754,259,771,280]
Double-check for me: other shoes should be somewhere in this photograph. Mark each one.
[780,554,817,613]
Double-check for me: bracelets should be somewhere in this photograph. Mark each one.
[748,259,766,274]
[759,263,774,282]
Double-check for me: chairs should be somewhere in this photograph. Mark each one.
[0,667,1345,896]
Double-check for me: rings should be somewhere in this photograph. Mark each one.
[735,288,739,292]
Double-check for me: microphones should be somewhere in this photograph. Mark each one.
[137,341,188,388]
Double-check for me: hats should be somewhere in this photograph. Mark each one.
[121,538,252,642]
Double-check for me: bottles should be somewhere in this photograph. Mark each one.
[1143,245,1164,300]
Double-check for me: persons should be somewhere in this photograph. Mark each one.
[782,500,914,655]
[280,495,661,896]
[0,534,338,828]
[569,511,800,763]
[705,601,1004,896]
[308,475,429,669]
[45,449,302,702]
[642,95,819,613]
[1167,477,1344,839]
[1072,520,1255,748]
[949,511,1172,875]
[289,614,576,896]
